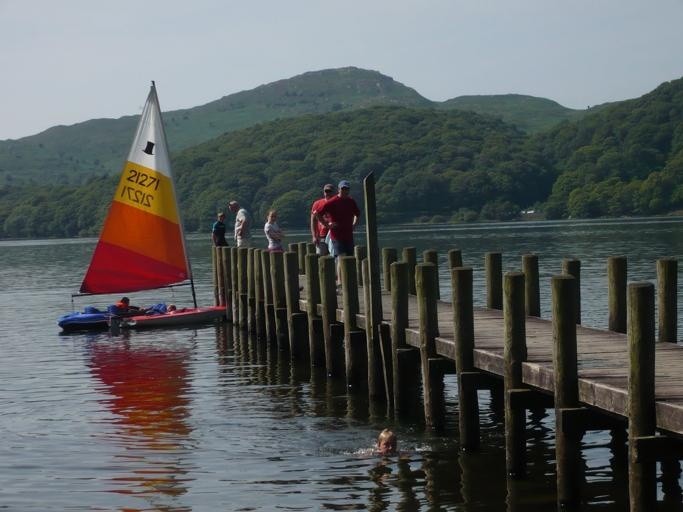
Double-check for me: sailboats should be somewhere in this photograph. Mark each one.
[56,80,226,331]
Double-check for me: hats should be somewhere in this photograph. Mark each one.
[323,184,337,192]
[338,180,351,189]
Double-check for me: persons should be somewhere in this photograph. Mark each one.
[310,180,361,294]
[116,296,130,309]
[264,209,284,250]
[376,428,398,454]
[167,303,176,313]
[211,200,254,248]
